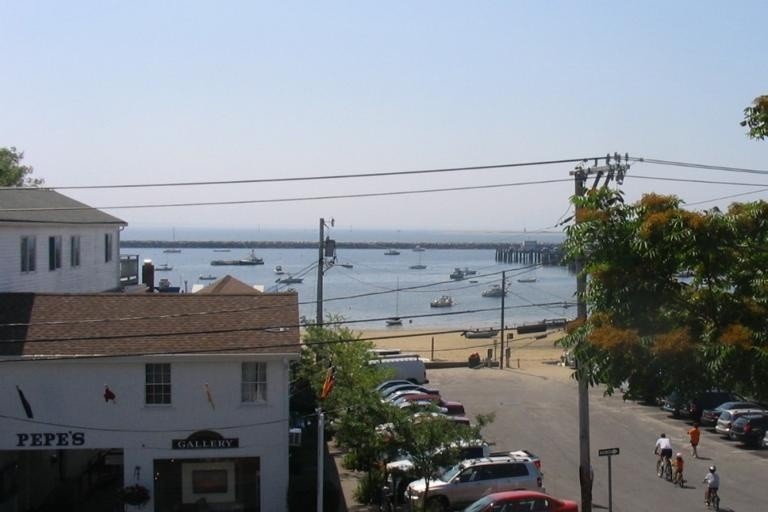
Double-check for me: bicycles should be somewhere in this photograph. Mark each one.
[702,479,720,511]
[655,452,673,481]
[671,467,683,488]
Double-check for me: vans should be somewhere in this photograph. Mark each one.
[363,348,402,356]
[359,352,430,387]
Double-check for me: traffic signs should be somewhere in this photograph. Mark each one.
[599,448,619,456]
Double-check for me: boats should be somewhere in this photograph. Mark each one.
[481,282,507,298]
[273,264,285,275]
[210,249,265,266]
[519,278,537,282]
[386,315,402,325]
[449,266,464,280]
[154,264,172,271]
[409,263,428,270]
[429,295,452,308]
[198,274,217,279]
[413,245,424,251]
[275,275,303,284]
[383,248,401,256]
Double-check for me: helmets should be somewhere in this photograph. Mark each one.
[675,452,683,458]
[709,465,716,471]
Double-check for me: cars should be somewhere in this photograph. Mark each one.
[660,387,768,448]
[329,379,579,512]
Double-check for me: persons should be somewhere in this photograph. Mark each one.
[686,422,701,458]
[669,452,685,485]
[702,465,720,508]
[653,430,673,472]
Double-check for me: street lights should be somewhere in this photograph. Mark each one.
[315,217,354,370]
